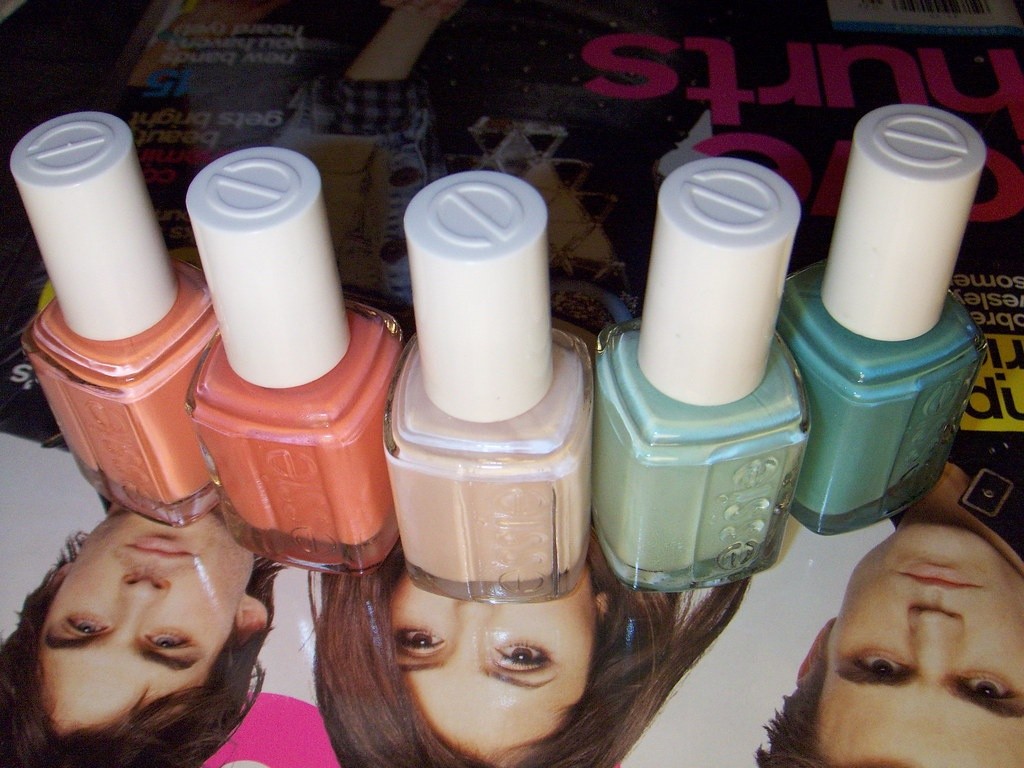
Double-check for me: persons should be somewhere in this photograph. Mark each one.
[754,446,1023,768]
[0,497,295,768]
[122,0,465,308]
[316,532,750,768]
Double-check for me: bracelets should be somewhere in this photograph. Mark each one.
[156,31,198,52]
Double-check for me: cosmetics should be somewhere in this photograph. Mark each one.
[8,99,990,587]
